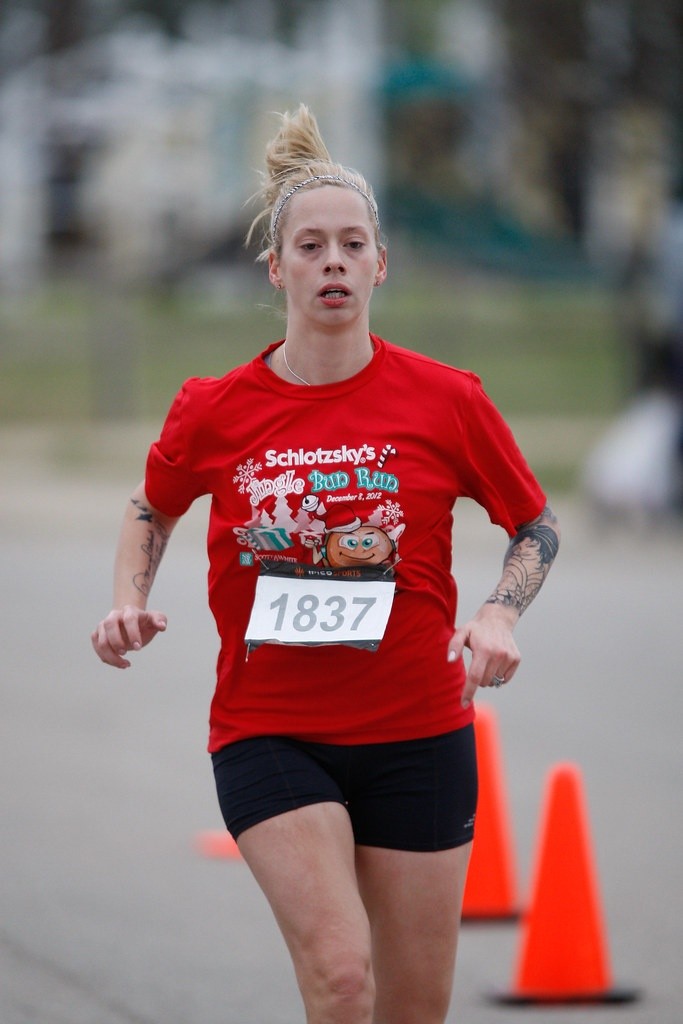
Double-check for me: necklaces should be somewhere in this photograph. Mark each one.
[283,341,310,386]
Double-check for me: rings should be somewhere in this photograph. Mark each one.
[492,676,504,686]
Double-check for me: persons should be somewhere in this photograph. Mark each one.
[92,114,559,1023]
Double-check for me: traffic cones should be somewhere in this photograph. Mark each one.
[483,759,643,1008]
[458,704,527,925]
